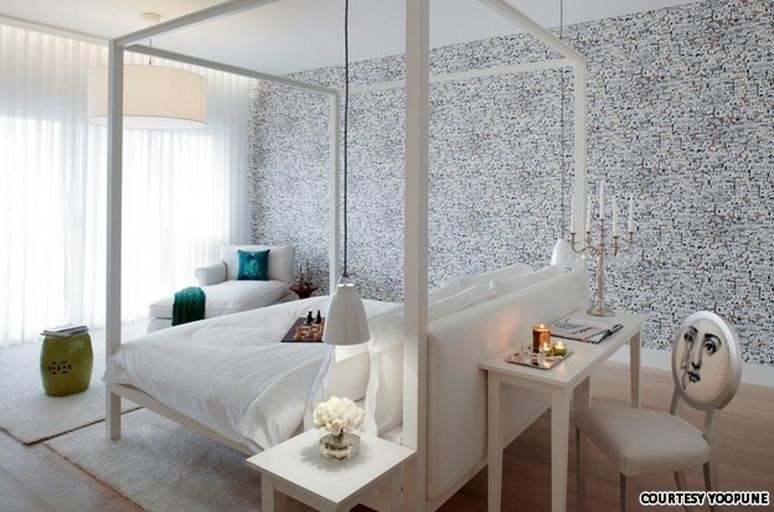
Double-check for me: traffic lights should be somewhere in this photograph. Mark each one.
[236,249,270,281]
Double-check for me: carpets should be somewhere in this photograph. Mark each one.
[0,318,316,511]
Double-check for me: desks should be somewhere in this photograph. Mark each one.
[478,303,645,512]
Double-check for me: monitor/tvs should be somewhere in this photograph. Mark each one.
[44,322,89,341]
[550,316,625,344]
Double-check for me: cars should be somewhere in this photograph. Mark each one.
[532,324,566,357]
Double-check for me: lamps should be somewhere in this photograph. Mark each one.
[548,1,576,268]
[568,308,744,512]
[320,1,371,348]
[144,245,295,333]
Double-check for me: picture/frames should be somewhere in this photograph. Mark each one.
[478,303,645,512]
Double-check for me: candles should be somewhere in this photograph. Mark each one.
[568,179,634,238]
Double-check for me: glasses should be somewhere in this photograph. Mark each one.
[320,1,371,348]
[548,1,576,268]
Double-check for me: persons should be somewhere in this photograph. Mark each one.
[682,324,721,386]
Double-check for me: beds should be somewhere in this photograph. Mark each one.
[105,265,589,443]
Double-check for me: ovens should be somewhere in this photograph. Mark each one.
[105,265,589,443]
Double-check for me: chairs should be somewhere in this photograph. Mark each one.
[568,308,744,512]
[144,245,295,333]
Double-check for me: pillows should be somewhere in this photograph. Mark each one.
[321,277,479,402]
[489,264,563,302]
[446,264,534,299]
[236,249,270,281]
[365,286,483,435]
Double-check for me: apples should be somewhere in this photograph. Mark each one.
[568,179,634,238]
